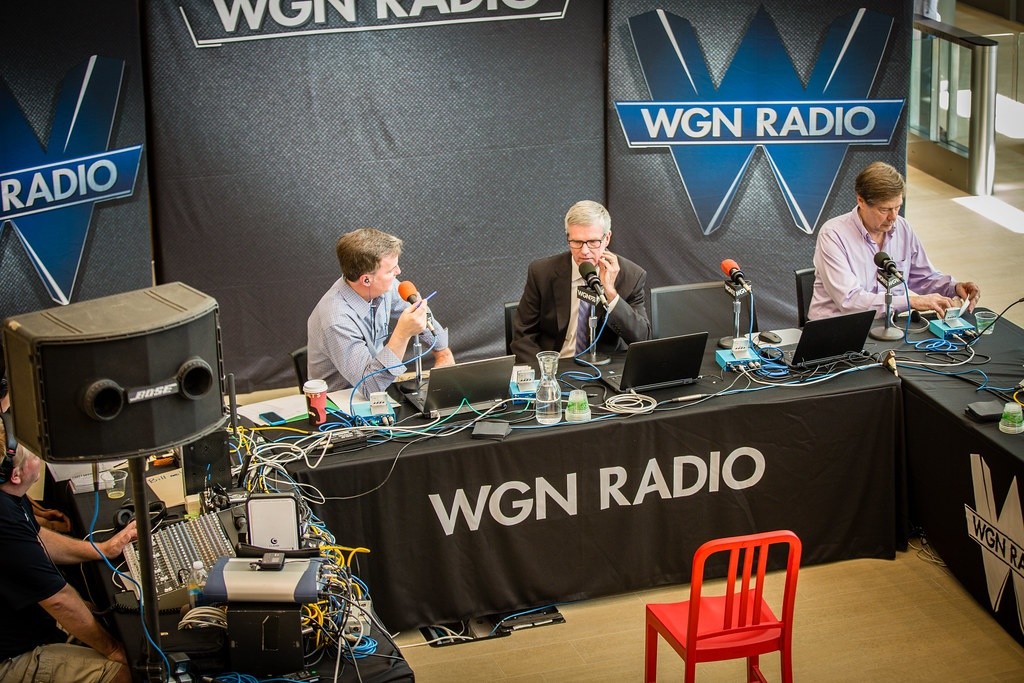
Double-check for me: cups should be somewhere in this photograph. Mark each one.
[999,402,1024,435]
[565,391,591,423]
[303,378,328,424]
[975,311,998,335]
[102,470,128,498]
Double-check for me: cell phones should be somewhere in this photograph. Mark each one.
[259,412,286,426]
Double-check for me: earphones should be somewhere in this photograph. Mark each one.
[365,279,370,283]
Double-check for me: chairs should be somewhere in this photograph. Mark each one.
[651,281,758,338]
[643,530,803,683]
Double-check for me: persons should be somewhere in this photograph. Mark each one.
[307,228,456,402]
[805,163,981,323]
[0,343,72,535]
[0,411,138,683]
[510,201,654,365]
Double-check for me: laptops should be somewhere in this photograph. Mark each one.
[594,331,708,394]
[763,309,877,370]
[404,355,516,419]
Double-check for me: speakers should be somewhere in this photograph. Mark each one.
[4,282,229,465]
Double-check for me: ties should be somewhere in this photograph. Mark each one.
[575,279,594,358]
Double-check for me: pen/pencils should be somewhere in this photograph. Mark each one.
[415,291,439,310]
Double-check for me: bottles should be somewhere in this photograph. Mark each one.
[534,350,562,425]
[187,560,208,611]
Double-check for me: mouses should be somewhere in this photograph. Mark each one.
[758,331,782,343]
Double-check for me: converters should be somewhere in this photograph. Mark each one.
[326,429,368,453]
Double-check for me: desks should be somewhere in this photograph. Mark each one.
[252,330,907,624]
[66,421,416,682]
[862,304,1023,637]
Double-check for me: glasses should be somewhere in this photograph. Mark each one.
[567,231,608,249]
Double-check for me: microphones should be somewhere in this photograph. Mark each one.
[579,262,608,308]
[874,252,905,282]
[721,258,752,294]
[398,281,437,337]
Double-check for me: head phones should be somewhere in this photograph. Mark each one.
[0,412,19,485]
[891,309,930,334]
[112,501,167,530]
